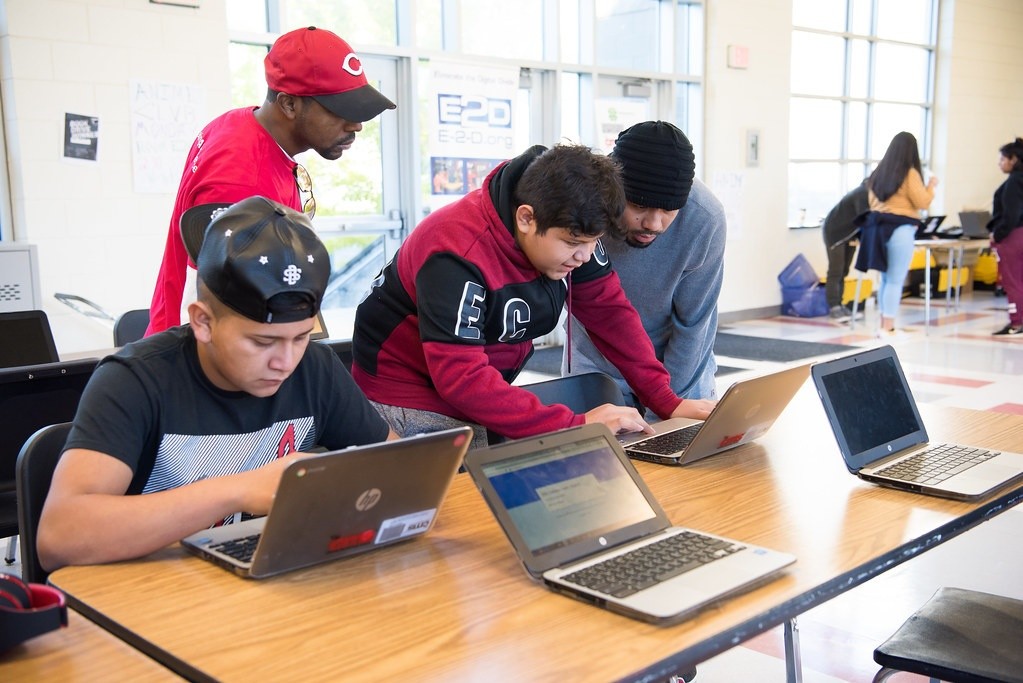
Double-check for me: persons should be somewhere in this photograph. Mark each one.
[36,194,400,572]
[142,26,396,337]
[433,166,448,194]
[560,121,727,417]
[985,137,1023,339]
[867,131,938,337]
[823,177,868,323]
[351,146,720,451]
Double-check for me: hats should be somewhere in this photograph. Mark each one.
[606,120,696,212]
[263,26,399,121]
[178,200,332,323]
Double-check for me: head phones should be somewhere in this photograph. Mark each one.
[0,572,70,657]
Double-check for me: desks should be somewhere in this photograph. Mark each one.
[849,236,989,335]
[49,405,1023,683]
[0,607,192,683]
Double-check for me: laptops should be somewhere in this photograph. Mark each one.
[958,211,991,236]
[462,423,797,626]
[914,216,948,239]
[179,426,474,579]
[613,361,817,465]
[810,344,1023,502]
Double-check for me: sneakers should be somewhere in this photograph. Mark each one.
[993,323,1023,339]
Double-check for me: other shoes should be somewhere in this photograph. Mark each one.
[828,305,862,322]
[875,326,909,340]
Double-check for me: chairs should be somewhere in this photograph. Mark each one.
[113,307,150,351]
[14,420,75,588]
[487,371,625,447]
[0,310,59,365]
[0,357,98,535]
[872,585,1023,681]
